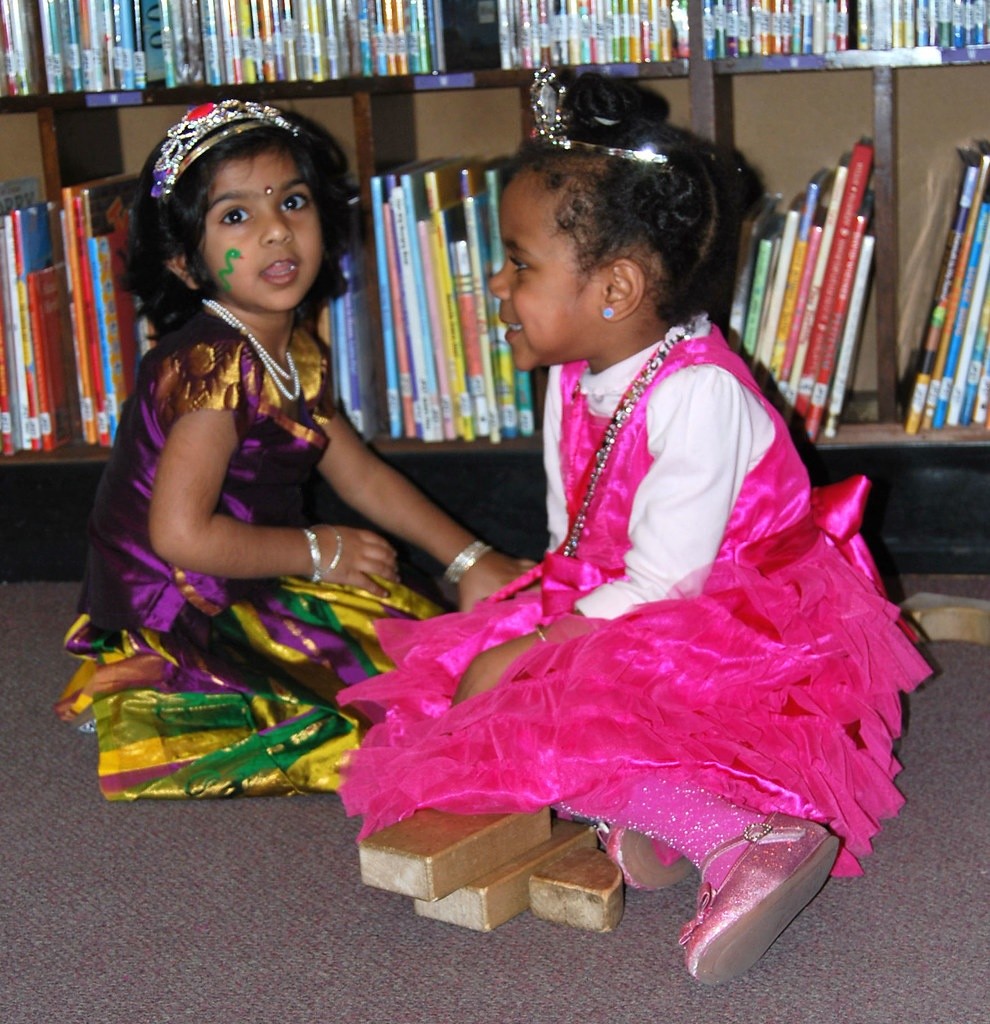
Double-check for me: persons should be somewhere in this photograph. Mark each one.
[334,75,934,988]
[53,99,539,723]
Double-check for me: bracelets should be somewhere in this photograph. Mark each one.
[299,525,324,586]
[320,524,345,580]
[442,540,494,585]
[535,623,547,642]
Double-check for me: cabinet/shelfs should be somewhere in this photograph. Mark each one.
[0,0,990,582]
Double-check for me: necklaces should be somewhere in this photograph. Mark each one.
[209,300,296,381]
[202,298,300,401]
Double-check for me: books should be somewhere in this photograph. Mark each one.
[0,0,990,461]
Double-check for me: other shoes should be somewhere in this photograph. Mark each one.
[607,826,693,891]
[679,811,840,985]
